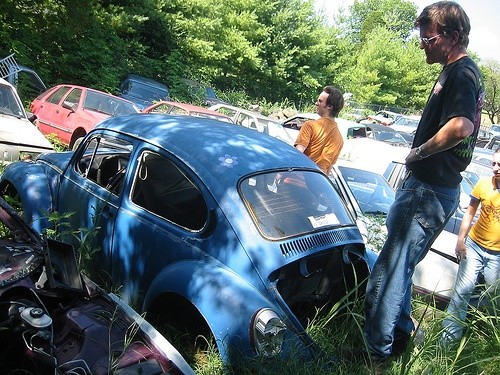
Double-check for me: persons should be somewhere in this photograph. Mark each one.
[440,147,500,348]
[341,1,484,365]
[274,86,344,204]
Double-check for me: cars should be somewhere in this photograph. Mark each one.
[28,84,141,153]
[205,102,499,313]
[117,73,169,110]
[0,78,56,167]
[140,101,236,125]
[1,112,379,366]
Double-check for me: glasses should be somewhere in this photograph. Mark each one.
[419,30,448,47]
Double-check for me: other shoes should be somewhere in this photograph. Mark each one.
[343,342,393,366]
[389,334,412,354]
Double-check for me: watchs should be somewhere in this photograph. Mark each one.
[416,147,427,158]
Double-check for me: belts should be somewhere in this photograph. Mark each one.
[404,169,413,180]
